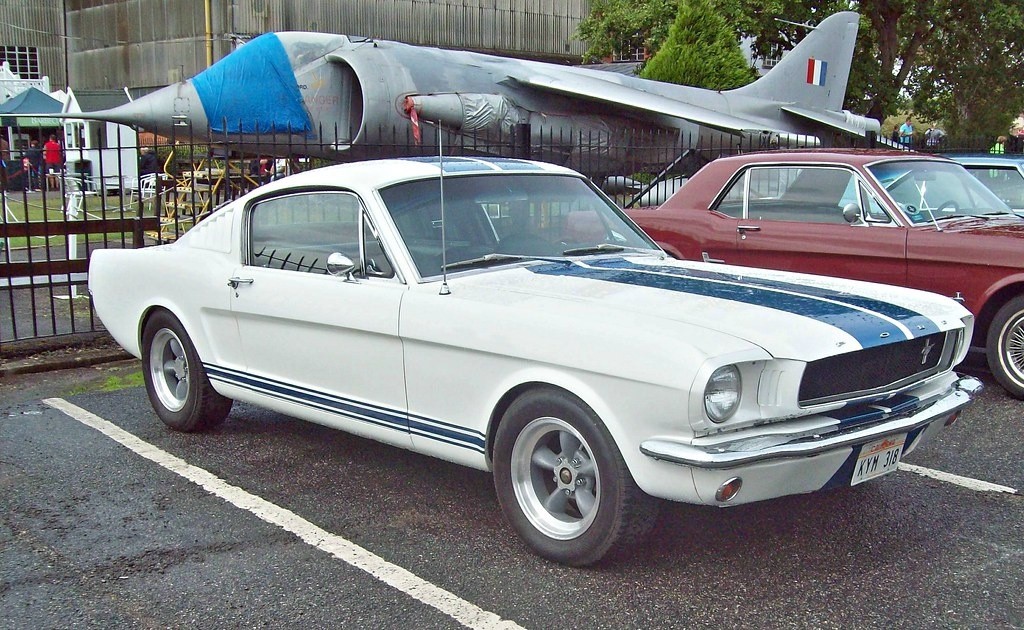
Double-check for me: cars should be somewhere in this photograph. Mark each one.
[840,157,1024,217]
[564,152,1024,403]
[88,156,986,569]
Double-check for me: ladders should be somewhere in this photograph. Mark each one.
[146,141,259,243]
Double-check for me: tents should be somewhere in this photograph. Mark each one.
[0,86,85,193]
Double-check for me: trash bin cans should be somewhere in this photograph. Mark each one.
[74,158,92,191]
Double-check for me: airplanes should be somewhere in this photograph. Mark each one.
[0,12,924,207]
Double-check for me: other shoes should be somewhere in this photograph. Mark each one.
[35,189,41,192]
[51,188,54,191]
[6,191,9,194]
[25,187,29,191]
[56,188,59,191]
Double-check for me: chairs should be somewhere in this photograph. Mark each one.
[57,176,102,212]
[130,173,168,211]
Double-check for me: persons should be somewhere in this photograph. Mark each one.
[900,117,914,148]
[0,135,10,193]
[925,123,945,148]
[891,124,905,144]
[140,147,155,189]
[43,135,63,191]
[249,159,290,192]
[22,140,43,192]
[989,136,1010,203]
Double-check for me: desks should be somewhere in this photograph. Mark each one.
[46,173,91,209]
[86,174,128,213]
